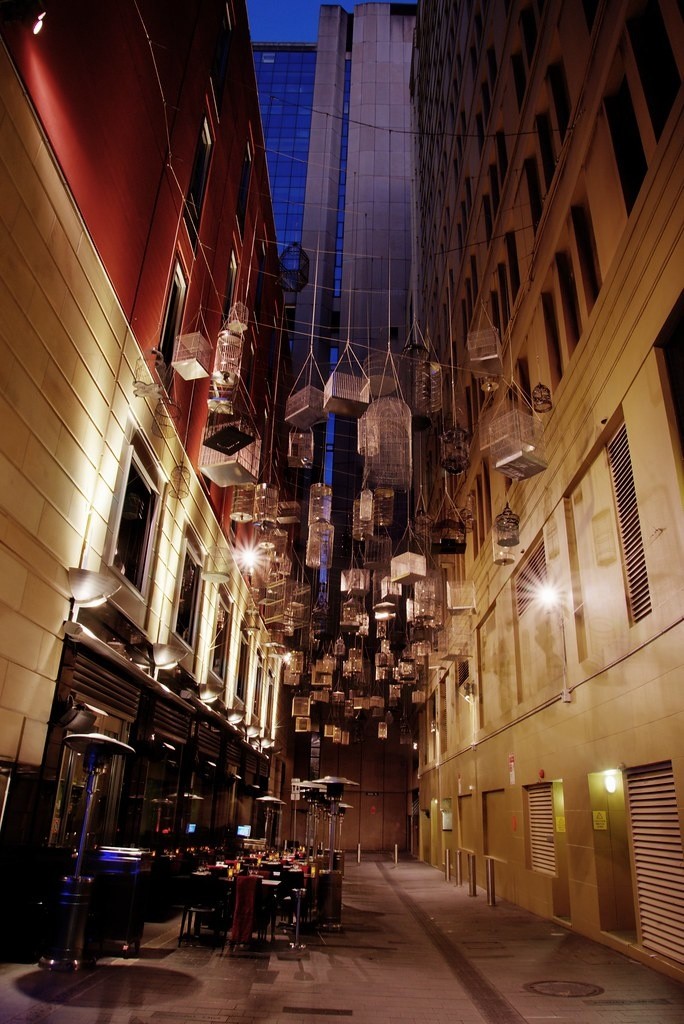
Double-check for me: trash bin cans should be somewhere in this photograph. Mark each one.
[38,877,93,970]
[334,850,345,879]
[324,850,329,870]
[316,870,344,931]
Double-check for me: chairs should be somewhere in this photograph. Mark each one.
[219,873,270,948]
[178,875,223,949]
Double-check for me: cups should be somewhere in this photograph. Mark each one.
[176,849,179,854]
[311,867,315,873]
[190,847,194,851]
[236,863,241,873]
[228,868,233,878]
[152,851,155,856]
[258,858,261,864]
[275,853,279,859]
[269,855,272,860]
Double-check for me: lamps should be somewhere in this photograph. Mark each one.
[261,738,274,748]
[246,725,263,737]
[153,642,188,669]
[256,775,362,931]
[38,731,135,971]
[68,567,123,607]
[227,708,247,723]
[199,683,226,702]
